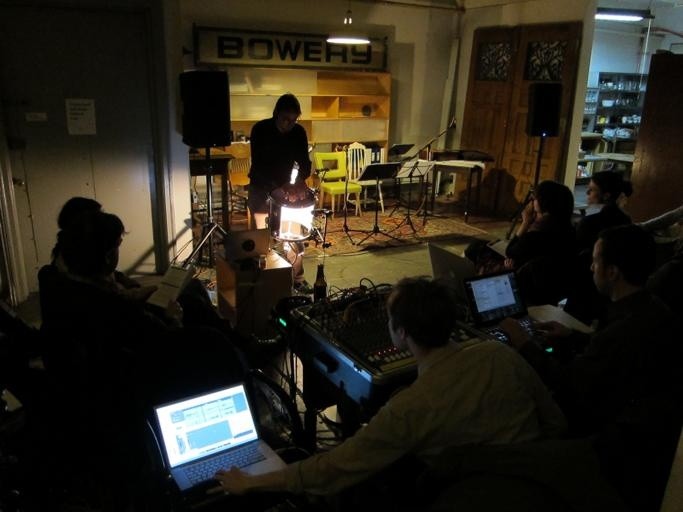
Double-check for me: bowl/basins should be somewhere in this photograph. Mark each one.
[601,100,614,107]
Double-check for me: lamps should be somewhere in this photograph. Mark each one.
[326,0,370,45]
[595,8,654,22]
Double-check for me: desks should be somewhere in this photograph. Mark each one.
[215,247,294,334]
[429,161,481,223]
[598,152,636,182]
[188,153,236,234]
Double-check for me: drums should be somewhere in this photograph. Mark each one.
[269,183,319,243]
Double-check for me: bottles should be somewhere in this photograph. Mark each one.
[313,265,327,302]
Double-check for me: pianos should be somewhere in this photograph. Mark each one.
[419,150,496,163]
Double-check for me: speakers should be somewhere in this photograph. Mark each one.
[178,69,231,148]
[525,83,562,136]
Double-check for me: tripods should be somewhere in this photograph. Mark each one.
[328,148,367,245]
[507,138,545,238]
[387,179,438,234]
[358,182,398,244]
[173,149,234,268]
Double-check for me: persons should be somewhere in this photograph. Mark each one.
[461,180,575,305]
[248,94,316,294]
[577,172,633,239]
[500,224,681,425]
[204,279,567,504]
[52,197,141,288]
[37,214,183,383]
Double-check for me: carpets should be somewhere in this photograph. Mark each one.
[302,197,488,258]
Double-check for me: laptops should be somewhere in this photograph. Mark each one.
[465,269,553,349]
[153,380,290,502]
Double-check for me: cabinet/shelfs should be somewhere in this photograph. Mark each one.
[581,71,648,134]
[206,65,391,204]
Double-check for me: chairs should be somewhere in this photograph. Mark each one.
[0,264,181,512]
[223,141,385,230]
